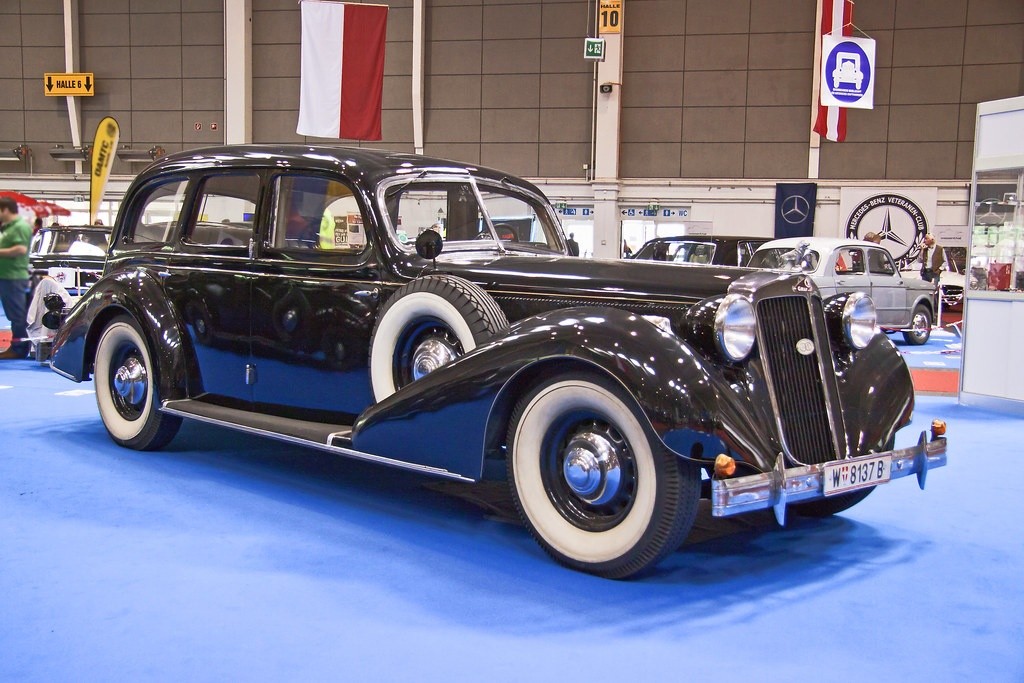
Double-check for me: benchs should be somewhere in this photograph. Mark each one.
[138,219,254,242]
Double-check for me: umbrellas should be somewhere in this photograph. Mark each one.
[0,191,70,217]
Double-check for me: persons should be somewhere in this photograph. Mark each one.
[320,208,335,249]
[0,197,32,356]
[918,234,947,287]
[93,219,105,243]
[567,233,579,257]
[863,233,886,271]
[624,239,632,253]
[32,218,43,234]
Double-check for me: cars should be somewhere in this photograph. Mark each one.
[48,143,947,580]
[27,226,112,340]
[895,249,965,311]
[626,236,937,345]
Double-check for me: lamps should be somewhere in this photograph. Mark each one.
[0,145,28,162]
[49,144,92,162]
[117,146,165,164]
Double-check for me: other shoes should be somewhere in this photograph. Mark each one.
[0,345,28,360]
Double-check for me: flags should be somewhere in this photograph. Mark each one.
[296,2,388,140]
